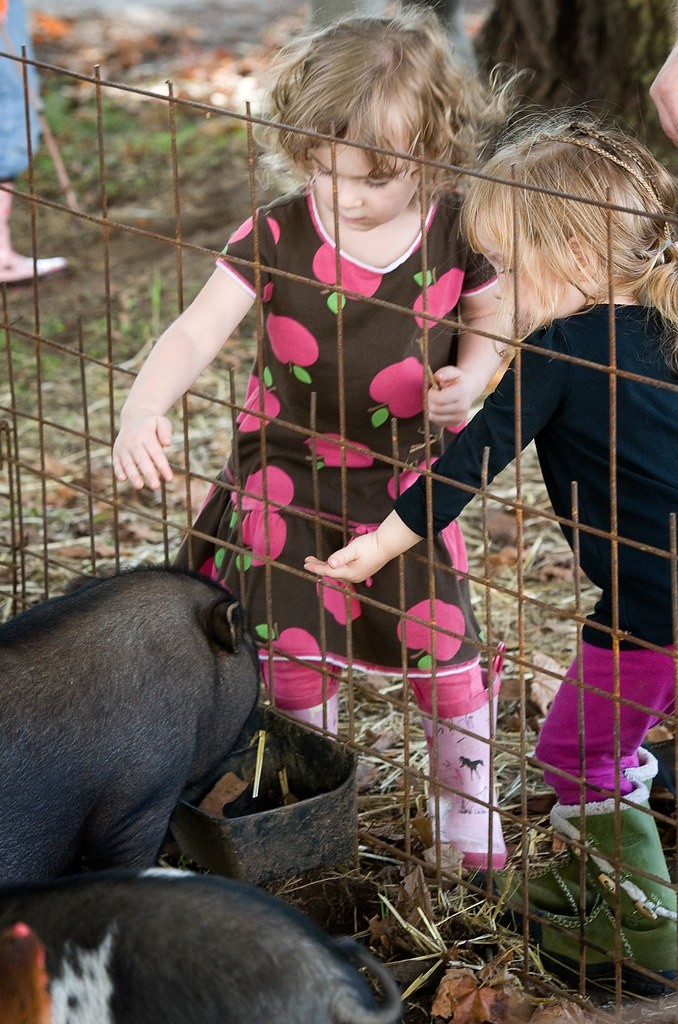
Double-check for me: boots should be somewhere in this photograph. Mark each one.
[272,666,340,739]
[421,670,507,870]
[474,747,658,933]
[530,779,678,996]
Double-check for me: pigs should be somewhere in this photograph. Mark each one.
[0,564,403,1024]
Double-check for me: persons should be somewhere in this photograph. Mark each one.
[104,6,509,880]
[305,119,678,1000]
[0,0,71,287]
